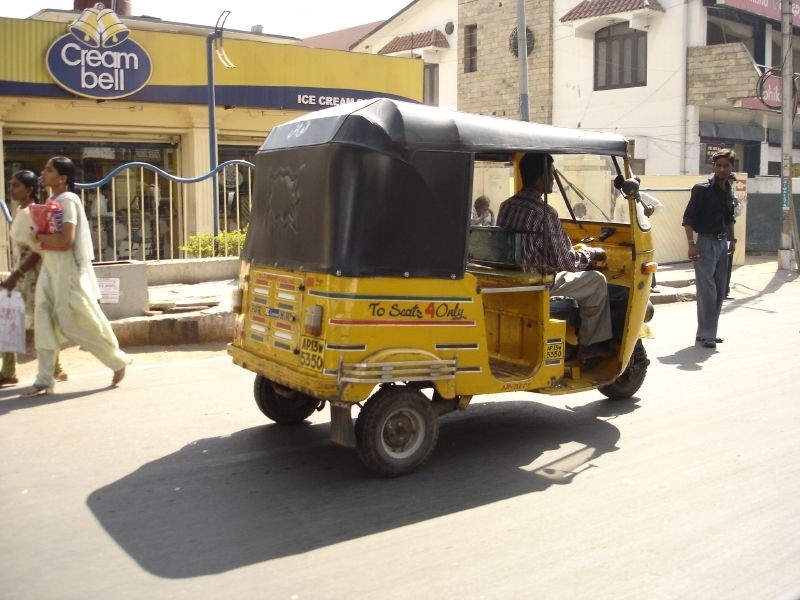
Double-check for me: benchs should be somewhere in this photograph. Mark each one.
[470,224,581,319]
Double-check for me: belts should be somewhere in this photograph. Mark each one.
[698,232,728,240]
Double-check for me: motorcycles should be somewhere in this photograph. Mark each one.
[227,98,661,478]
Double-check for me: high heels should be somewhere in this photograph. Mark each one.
[113,356,133,384]
[22,385,54,399]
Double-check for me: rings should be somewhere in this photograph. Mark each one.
[693,256,696,257]
[697,255,699,256]
[690,257,692,258]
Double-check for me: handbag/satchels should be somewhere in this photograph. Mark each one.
[0,290,26,353]
[29,200,68,250]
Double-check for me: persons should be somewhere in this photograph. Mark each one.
[470,196,495,227]
[681,149,738,347]
[497,152,613,359]
[18,155,133,398]
[0,170,68,384]
[90,184,114,247]
[614,177,659,292]
[573,203,587,220]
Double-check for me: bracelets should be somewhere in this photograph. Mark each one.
[731,239,737,242]
[689,240,693,242]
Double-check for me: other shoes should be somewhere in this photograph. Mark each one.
[577,344,615,359]
[705,339,716,347]
[0,373,18,386]
[49,371,68,381]
[696,336,722,342]
